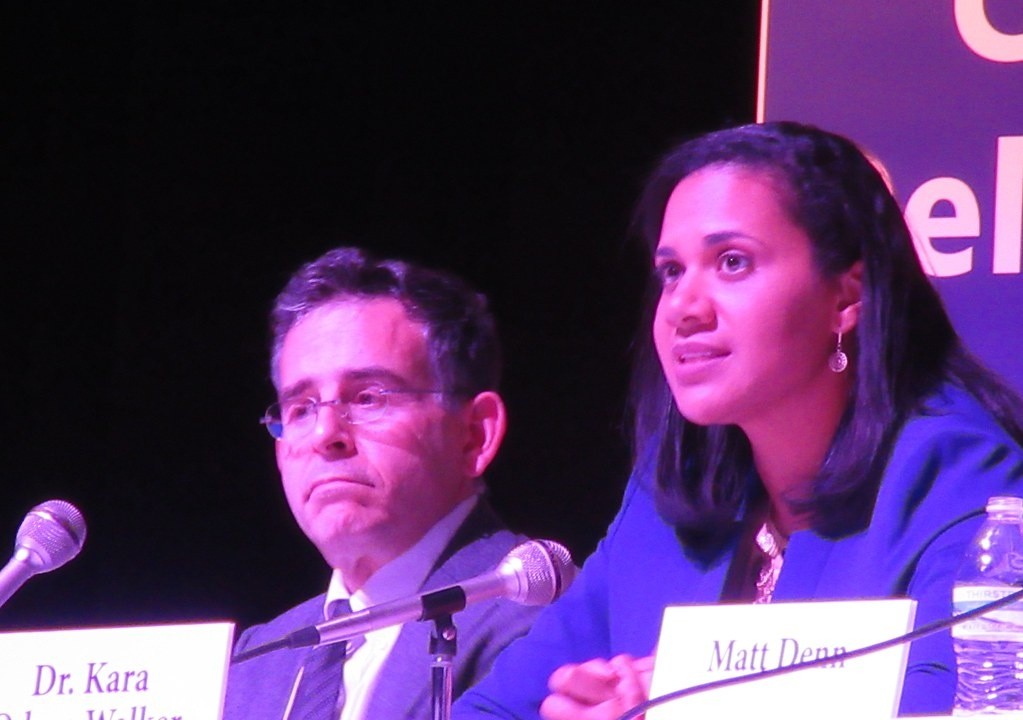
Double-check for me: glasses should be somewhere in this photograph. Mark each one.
[258,382,461,442]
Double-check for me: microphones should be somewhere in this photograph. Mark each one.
[0,500,87,607]
[286,540,576,647]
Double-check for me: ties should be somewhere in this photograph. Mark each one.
[287,599,351,720]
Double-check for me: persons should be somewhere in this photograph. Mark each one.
[447,122,1023,720]
[221,246,582,720]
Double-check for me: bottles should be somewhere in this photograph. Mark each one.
[947,497,1023,720]
